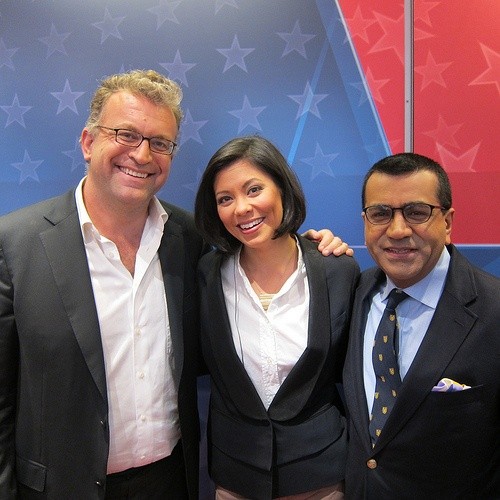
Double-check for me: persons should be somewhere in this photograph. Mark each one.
[194,137,360,500]
[0,71,353,500]
[337,152,500,500]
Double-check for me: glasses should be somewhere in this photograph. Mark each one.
[95,124,177,155]
[363,202,448,225]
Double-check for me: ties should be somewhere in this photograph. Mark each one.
[368,288,410,450]
